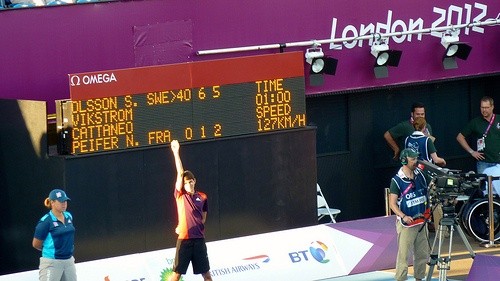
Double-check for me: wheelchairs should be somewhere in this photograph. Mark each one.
[458,172,500,244]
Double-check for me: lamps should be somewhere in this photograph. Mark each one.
[439,22,472,70]
[370,31,403,79]
[305,40,338,86]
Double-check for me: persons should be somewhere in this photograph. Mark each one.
[389,149,431,281]
[457,96,500,244]
[170,140,214,281]
[384,102,446,264]
[32,189,77,281]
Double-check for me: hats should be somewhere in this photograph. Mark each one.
[399,148,419,159]
[49,189,70,202]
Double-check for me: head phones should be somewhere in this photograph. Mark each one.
[400,150,408,165]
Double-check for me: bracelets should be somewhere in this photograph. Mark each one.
[403,215,406,218]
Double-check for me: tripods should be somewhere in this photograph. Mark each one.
[426,201,475,281]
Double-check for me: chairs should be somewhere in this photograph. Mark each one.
[316,183,341,223]
